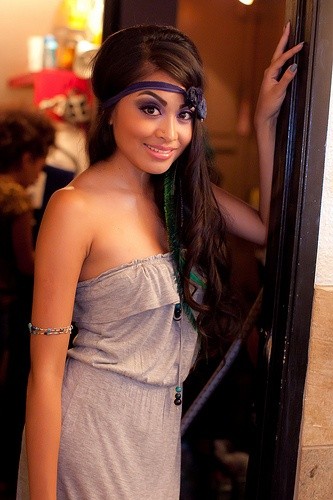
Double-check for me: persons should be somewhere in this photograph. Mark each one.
[0,103,59,279]
[12,20,306,499]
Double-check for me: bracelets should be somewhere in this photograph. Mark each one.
[28,323,74,337]
